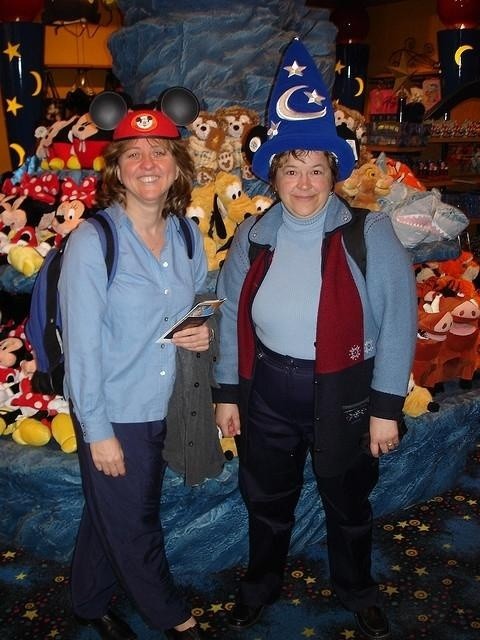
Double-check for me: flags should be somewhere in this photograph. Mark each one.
[433,26,480,107]
[333,40,373,160]
[1,20,47,171]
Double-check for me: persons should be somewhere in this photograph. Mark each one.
[53,107,221,638]
[214,140,421,639]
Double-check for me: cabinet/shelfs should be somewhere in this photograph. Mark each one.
[362,136,478,234]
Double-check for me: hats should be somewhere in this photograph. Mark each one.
[89,86,200,142]
[245,37,359,184]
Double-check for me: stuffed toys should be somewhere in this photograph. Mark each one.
[178,107,275,270]
[0,92,113,456]
[0,91,480,460]
[337,153,480,417]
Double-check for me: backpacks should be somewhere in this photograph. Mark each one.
[24,210,195,372]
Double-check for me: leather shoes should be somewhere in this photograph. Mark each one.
[354,606,391,639]
[228,602,272,628]
[164,616,208,640]
[74,609,138,640]
